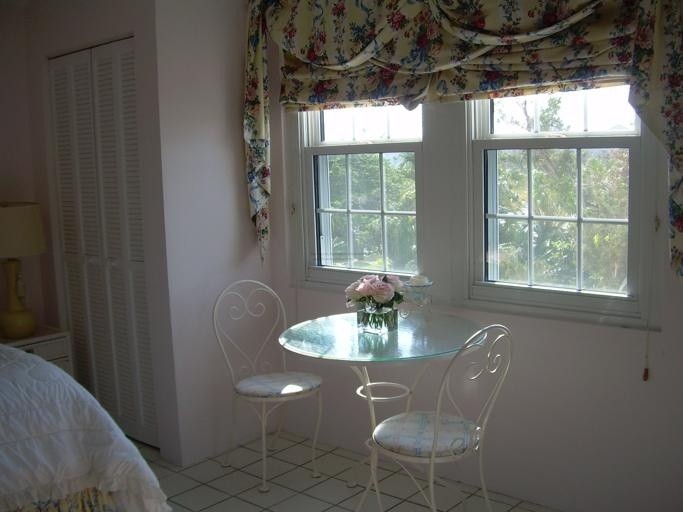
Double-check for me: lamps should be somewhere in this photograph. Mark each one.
[0,202,48,339]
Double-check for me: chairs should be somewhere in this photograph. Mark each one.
[367,325,514,510]
[212,279,322,492]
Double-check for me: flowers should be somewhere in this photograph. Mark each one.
[345,274,406,328]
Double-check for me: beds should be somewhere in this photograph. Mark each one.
[0,326,167,512]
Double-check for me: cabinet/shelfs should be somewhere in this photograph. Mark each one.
[29,1,276,468]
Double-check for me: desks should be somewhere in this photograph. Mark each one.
[279,311,486,511]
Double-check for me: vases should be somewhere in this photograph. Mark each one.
[357,308,397,331]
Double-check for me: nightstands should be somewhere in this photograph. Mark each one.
[1,325,74,380]
